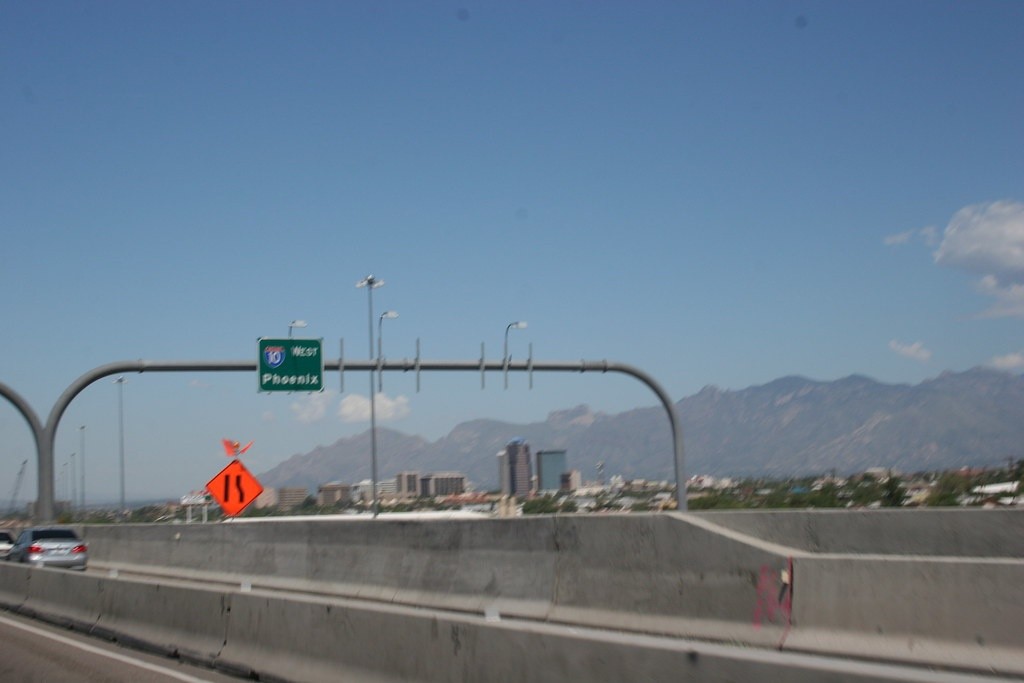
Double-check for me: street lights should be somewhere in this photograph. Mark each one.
[112,375,127,511]
[355,271,384,516]
[80,421,86,508]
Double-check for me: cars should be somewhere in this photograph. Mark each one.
[8,525,89,573]
[0,528,17,560]
